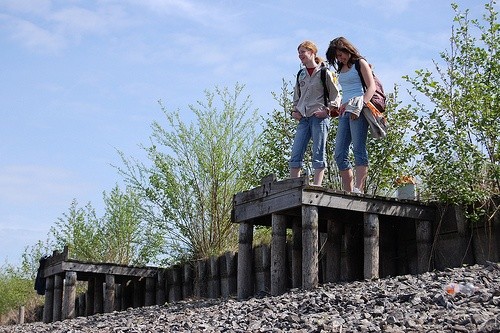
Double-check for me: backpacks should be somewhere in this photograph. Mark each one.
[355,57,386,112]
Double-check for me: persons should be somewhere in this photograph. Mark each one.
[290,40,342,187]
[326,36,378,194]
